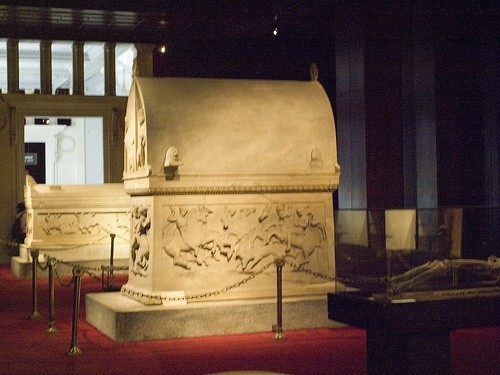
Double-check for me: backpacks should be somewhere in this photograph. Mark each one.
[11,211,27,240]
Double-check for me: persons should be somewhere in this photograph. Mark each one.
[11,202,27,246]
